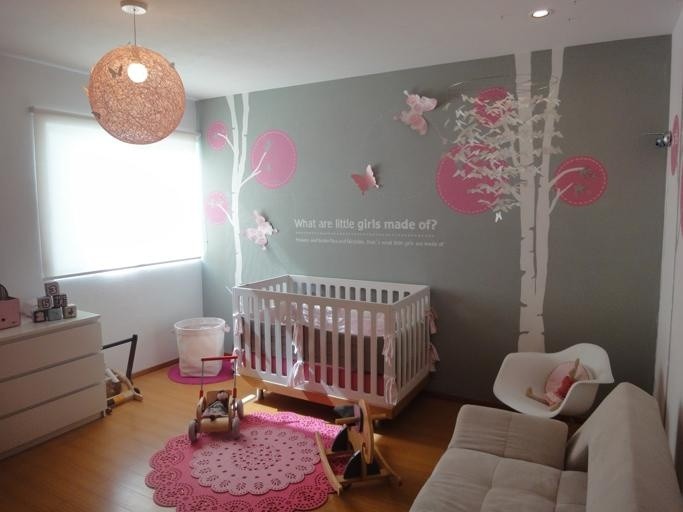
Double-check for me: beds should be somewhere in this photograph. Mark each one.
[233,274,431,421]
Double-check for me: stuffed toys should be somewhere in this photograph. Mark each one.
[201,389,229,422]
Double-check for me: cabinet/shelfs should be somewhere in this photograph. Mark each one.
[1,306,109,463]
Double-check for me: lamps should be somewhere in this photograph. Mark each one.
[88,0,187,144]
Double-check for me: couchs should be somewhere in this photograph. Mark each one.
[409,380,676,512]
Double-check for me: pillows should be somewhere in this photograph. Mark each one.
[545,362,594,404]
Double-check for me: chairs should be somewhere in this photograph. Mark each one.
[492,342,615,419]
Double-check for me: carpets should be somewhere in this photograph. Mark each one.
[145,410,353,512]
[167,361,234,384]
